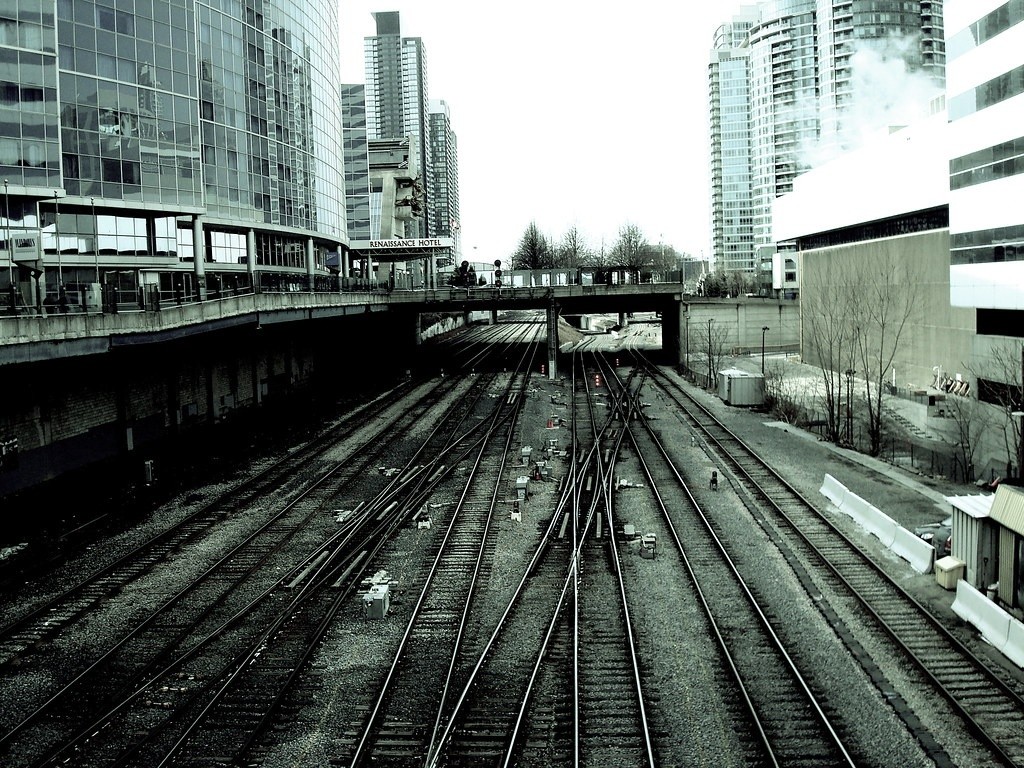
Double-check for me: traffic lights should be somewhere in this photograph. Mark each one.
[494,260,502,288]
